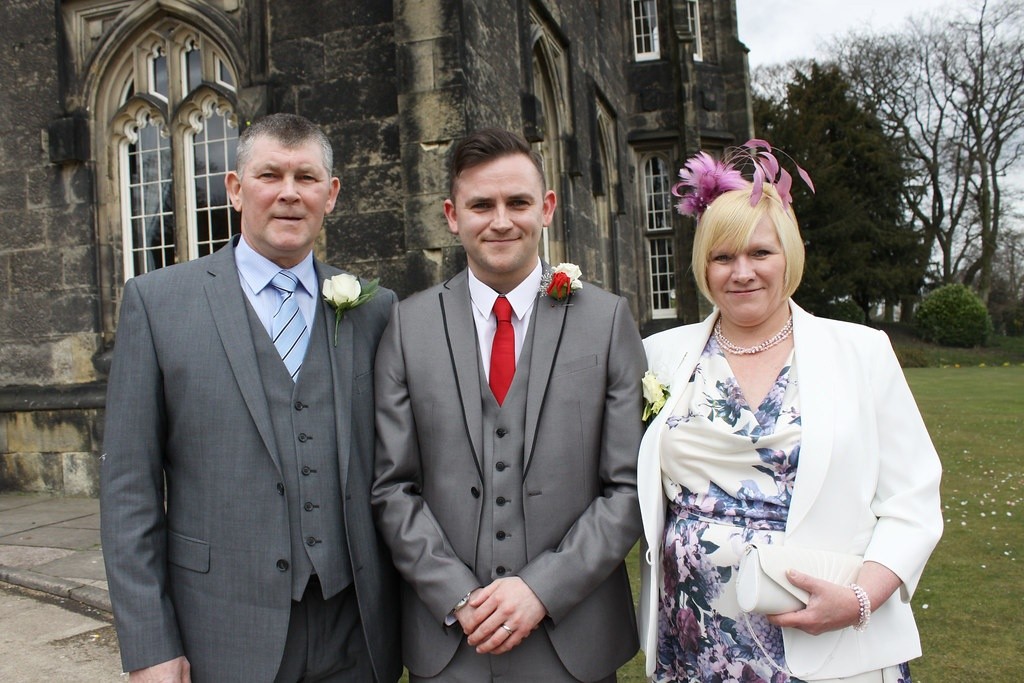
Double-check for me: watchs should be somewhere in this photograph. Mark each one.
[455,592,470,611]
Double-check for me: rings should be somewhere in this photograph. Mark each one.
[501,624,512,635]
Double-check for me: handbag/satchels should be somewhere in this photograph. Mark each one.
[736,543,864,616]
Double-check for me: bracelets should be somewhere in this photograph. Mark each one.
[848,583,871,632]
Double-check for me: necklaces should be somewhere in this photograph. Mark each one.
[714,315,793,355]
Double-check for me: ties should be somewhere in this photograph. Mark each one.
[270,270,310,382]
[488,296,516,407]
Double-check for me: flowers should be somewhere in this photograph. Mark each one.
[668,135,814,218]
[548,261,584,304]
[639,370,669,425]
[321,272,378,343]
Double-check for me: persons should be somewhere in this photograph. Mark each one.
[100,113,400,683]
[637,139,946,683]
[372,127,652,683]
